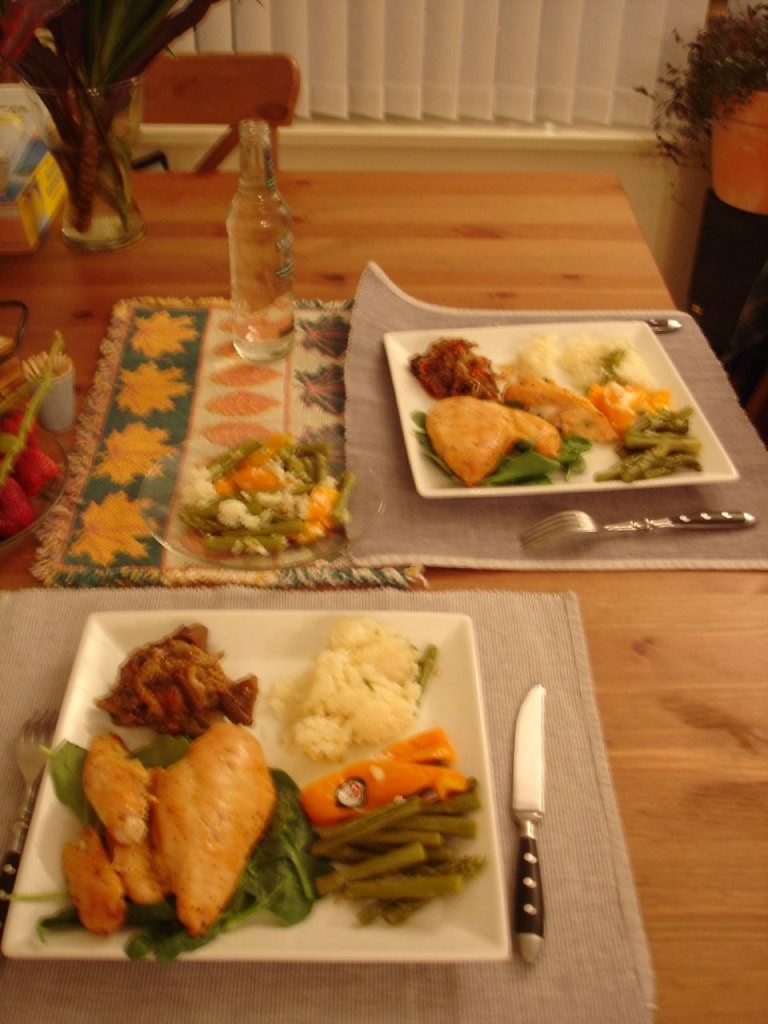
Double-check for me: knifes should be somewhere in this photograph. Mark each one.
[512,683,545,964]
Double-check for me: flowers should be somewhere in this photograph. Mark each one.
[0,0,264,232]
[634,2,768,214]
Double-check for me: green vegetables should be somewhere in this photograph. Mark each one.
[0,735,317,960]
[411,403,591,487]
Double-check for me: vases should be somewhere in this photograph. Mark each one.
[713,88,768,213]
[21,69,146,249]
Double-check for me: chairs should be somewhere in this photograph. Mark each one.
[143,52,299,170]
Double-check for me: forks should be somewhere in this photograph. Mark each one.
[517,509,757,548]
[1,709,60,938]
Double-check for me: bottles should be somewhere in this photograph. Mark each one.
[226,120,297,364]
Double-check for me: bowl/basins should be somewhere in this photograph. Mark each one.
[0,425,68,557]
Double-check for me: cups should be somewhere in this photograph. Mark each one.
[27,361,75,432]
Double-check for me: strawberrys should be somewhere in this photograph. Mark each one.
[0,411,59,536]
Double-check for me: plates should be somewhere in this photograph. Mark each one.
[1,608,511,963]
[136,423,384,571]
[384,320,741,499]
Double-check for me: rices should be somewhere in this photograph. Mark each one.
[520,334,654,386]
[271,616,438,763]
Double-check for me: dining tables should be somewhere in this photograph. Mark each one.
[1,169,767,1024]
